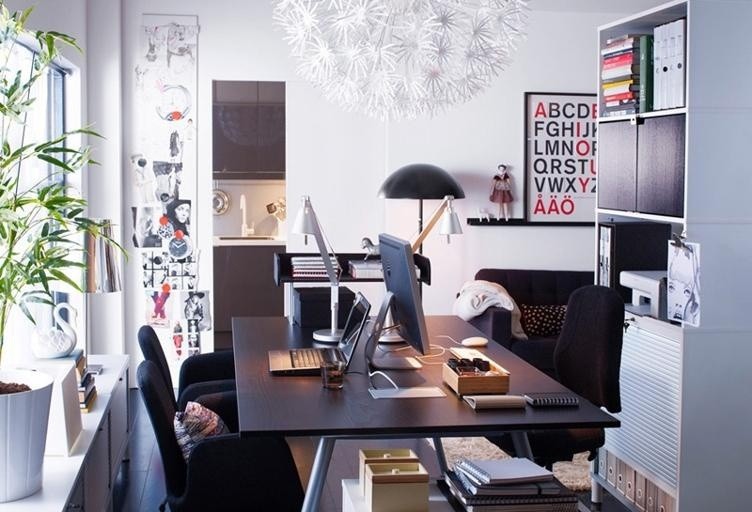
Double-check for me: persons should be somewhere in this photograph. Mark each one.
[129,118,205,361]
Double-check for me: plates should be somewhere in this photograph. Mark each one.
[590,1,752,509]
[1,352,131,511]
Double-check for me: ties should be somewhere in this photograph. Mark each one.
[362,233,430,369]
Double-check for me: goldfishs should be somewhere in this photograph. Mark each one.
[22,303,78,360]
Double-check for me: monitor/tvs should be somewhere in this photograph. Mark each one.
[37,349,98,413]
[290,255,339,279]
[601,15,687,118]
[436,457,581,512]
[521,391,580,407]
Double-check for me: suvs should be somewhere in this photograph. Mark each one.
[0,368,54,503]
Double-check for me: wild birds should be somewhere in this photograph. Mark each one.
[268,291,371,375]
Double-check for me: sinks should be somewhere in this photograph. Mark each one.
[453,269,594,381]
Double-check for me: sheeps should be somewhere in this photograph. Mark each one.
[240,194,255,236]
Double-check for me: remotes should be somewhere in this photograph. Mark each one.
[462,336,488,346]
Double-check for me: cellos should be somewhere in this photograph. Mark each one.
[619,269,670,322]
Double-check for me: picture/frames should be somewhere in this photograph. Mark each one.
[272,250,430,317]
[229,313,622,511]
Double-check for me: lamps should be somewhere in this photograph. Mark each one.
[484,282,626,472]
[134,321,305,511]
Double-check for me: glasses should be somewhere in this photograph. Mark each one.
[271,0,533,123]
[293,196,349,343]
[364,163,466,344]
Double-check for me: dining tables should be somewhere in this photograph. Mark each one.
[1,2,131,501]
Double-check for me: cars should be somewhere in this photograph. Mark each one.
[320,360,346,389]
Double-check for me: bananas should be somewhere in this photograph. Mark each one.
[449,347,511,375]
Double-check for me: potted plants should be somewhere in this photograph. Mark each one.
[523,91,596,226]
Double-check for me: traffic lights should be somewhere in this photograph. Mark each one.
[519,303,567,338]
